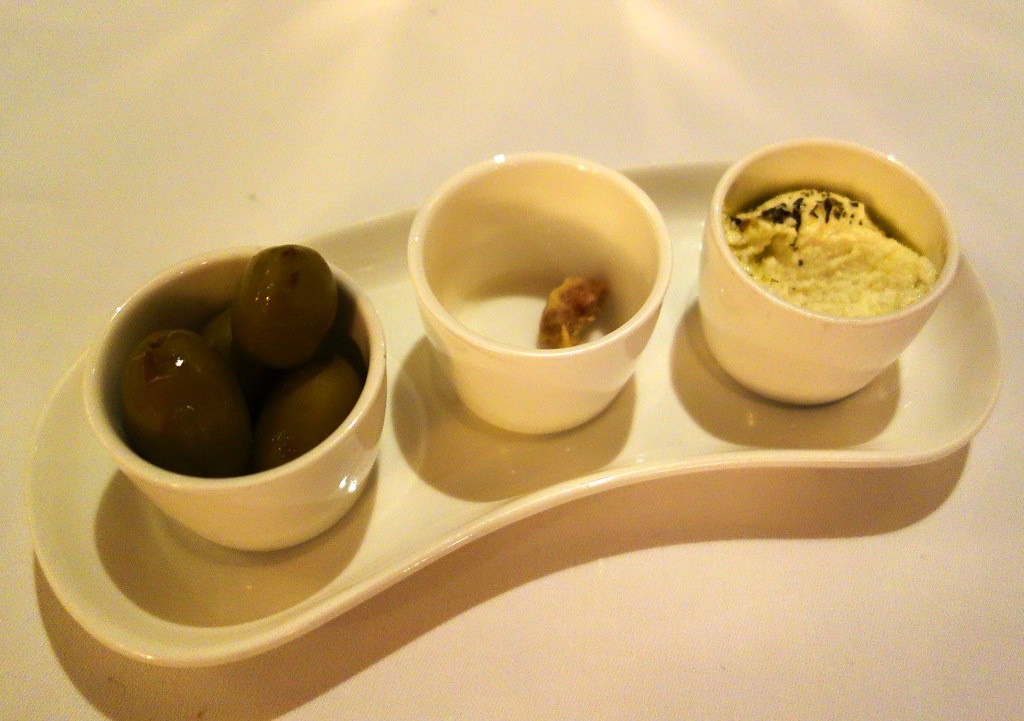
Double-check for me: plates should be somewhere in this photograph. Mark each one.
[28,156,1005,673]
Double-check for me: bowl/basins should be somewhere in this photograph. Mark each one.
[405,151,674,435]
[697,138,960,409]
[83,246,387,552]
